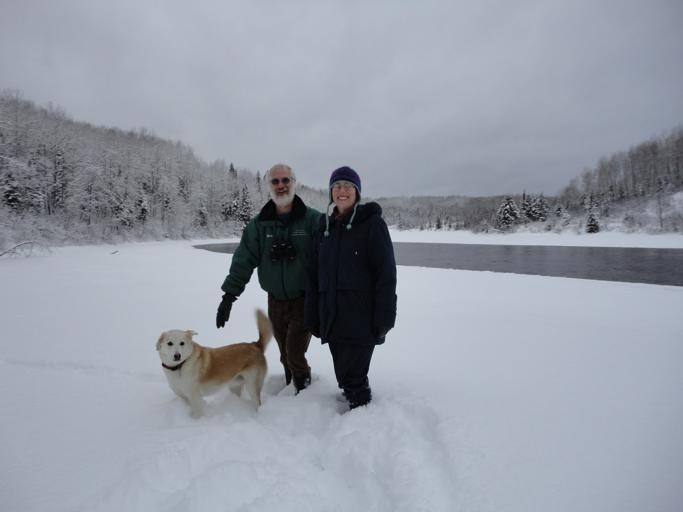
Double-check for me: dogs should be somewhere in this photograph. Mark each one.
[156,309,274,419]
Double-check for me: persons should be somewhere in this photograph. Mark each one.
[305,166,398,411]
[214,165,331,395]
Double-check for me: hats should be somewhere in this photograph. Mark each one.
[323,166,361,237]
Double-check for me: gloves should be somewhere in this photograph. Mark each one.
[216,295,237,328]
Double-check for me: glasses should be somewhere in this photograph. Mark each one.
[331,182,354,191]
[271,178,289,185]
